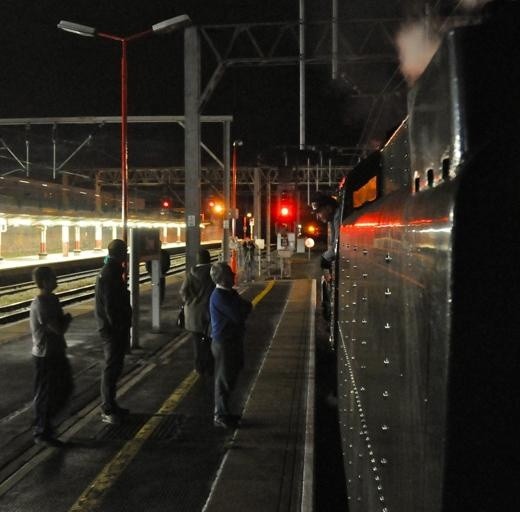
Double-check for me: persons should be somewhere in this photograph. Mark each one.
[29,265,74,448]
[94,239,132,425]
[241,240,255,283]
[146,240,170,308]
[178,247,253,430]
[309,191,341,282]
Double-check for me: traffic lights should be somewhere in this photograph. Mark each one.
[278,185,294,222]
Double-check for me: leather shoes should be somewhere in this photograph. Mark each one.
[101,408,130,425]
[214,413,241,429]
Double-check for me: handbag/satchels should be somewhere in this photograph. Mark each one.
[177,309,184,329]
[201,335,210,352]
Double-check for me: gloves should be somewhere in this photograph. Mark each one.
[321,256,331,268]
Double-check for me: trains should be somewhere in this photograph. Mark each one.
[321,16,520,510]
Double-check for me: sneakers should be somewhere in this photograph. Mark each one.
[35,431,61,447]
[194,367,213,375]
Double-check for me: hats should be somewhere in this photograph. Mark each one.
[311,193,330,214]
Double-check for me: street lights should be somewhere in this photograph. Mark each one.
[50,14,189,276]
[231,139,245,283]
